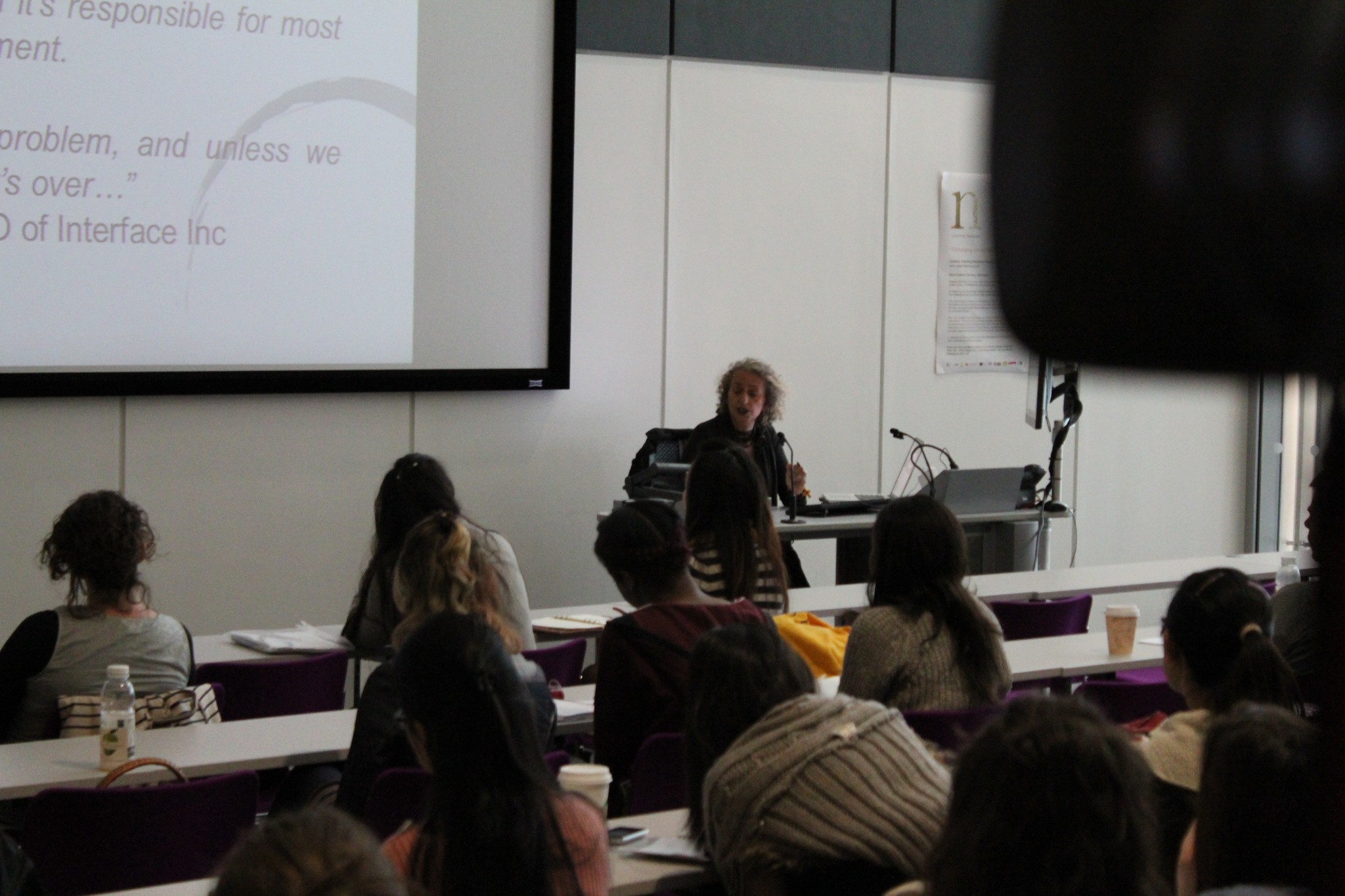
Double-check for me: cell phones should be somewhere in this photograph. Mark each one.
[606,825,646,844]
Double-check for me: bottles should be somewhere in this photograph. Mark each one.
[100,664,136,773]
[1274,557,1302,594]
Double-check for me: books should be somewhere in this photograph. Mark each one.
[630,827,712,869]
[527,609,615,635]
[233,621,357,657]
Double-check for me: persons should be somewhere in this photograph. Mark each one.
[340,452,538,667]
[334,509,560,835]
[2,485,196,744]
[831,492,1013,715]
[681,441,791,615]
[377,606,616,896]
[682,356,813,589]
[692,564,1345,894]
[211,802,403,896]
[590,496,816,816]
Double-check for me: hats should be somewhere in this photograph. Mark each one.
[701,695,956,896]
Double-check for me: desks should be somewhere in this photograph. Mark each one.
[0,498,1316,896]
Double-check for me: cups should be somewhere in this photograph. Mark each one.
[555,763,613,823]
[1104,605,1139,656]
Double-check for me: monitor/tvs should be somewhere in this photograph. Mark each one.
[1024,348,1048,430]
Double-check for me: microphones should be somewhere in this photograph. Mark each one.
[890,427,936,502]
[776,432,804,524]
[910,444,957,502]
